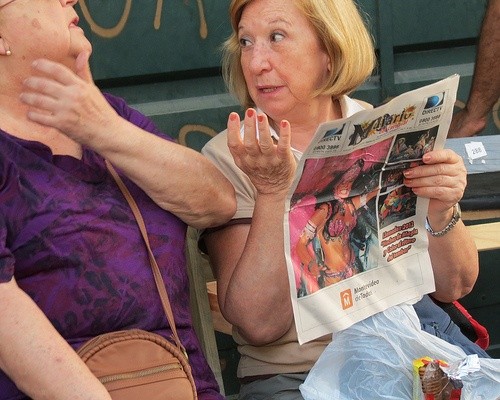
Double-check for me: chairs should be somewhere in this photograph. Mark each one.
[184,225,238,400]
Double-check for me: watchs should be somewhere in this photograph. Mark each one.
[424,201,463,238]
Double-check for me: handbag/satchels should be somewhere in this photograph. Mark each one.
[75,329,198,400]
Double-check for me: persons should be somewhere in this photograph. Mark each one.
[203,0,500,400]
[0,1,239,400]
